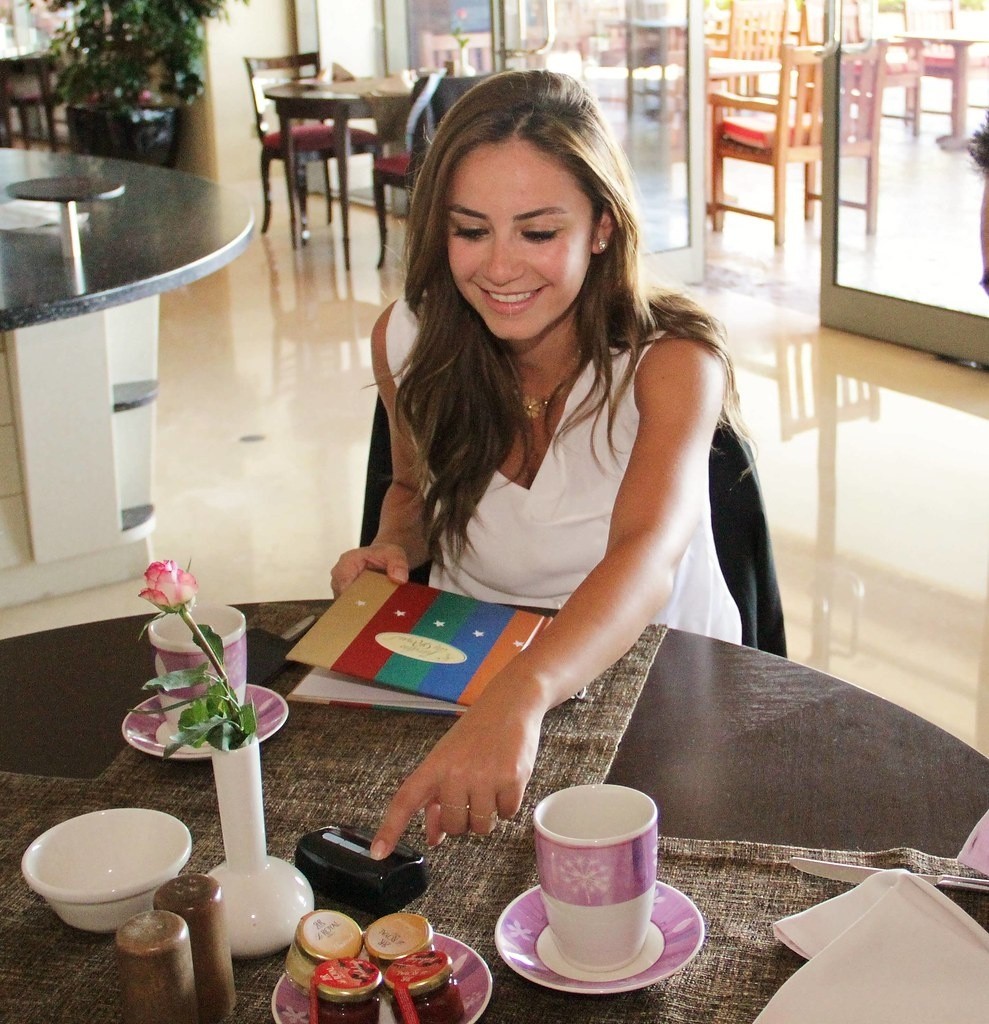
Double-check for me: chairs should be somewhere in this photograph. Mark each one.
[244,52,377,233]
[697,0,957,246]
[372,73,497,270]
[0,54,66,152]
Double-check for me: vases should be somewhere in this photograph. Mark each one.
[207,738,315,958]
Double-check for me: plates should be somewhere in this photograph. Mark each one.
[121,683,288,759]
[495,882,704,993]
[271,932,493,1024]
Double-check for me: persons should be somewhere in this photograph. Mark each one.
[331,69,744,861]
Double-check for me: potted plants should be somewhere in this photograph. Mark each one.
[29,0,248,168]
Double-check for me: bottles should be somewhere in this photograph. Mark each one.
[114,874,237,1024]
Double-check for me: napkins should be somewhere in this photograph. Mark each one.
[752,868,989,1024]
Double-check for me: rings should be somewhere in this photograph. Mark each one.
[470,810,498,822]
[441,802,470,810]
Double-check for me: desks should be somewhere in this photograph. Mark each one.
[897,29,989,149]
[0,600,989,1024]
[265,76,420,272]
[639,57,797,213]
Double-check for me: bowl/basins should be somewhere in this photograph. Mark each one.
[21,808,192,933]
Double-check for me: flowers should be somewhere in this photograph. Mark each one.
[126,555,257,761]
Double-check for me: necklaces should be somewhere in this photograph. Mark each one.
[512,347,583,419]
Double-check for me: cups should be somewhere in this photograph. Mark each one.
[533,785,657,972]
[148,604,249,736]
